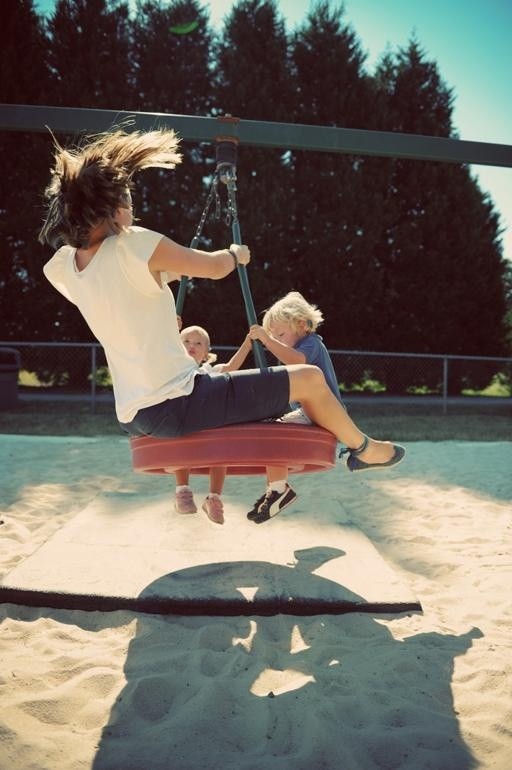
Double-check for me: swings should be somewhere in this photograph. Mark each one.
[131,161,336,475]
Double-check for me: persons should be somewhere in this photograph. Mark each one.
[245,286,347,523]
[37,114,409,475]
[175,315,253,525]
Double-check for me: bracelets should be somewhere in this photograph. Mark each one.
[226,247,239,270]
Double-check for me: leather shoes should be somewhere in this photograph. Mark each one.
[339,435,404,471]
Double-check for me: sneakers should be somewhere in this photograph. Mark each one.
[175,491,197,514]
[247,483,296,523]
[202,496,224,523]
